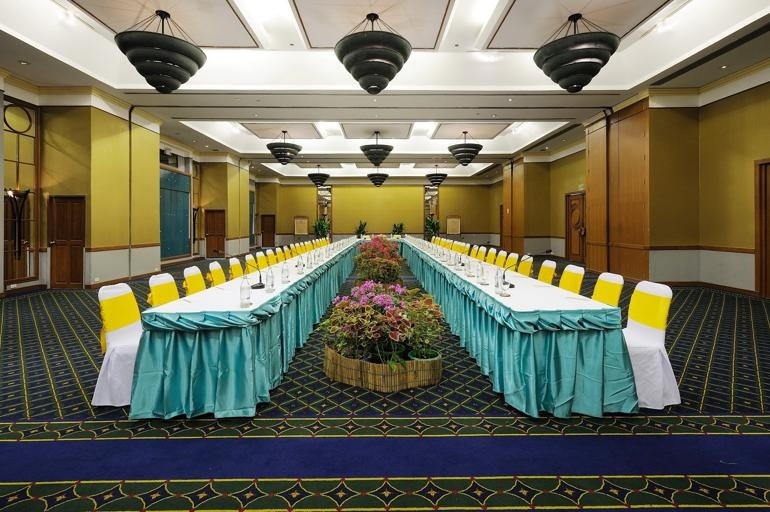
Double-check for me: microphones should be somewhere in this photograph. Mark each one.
[503,248,552,287]
[286,245,304,267]
[457,241,490,265]
[213,249,264,290]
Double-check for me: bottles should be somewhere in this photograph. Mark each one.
[237,234,359,308]
[403,233,513,298]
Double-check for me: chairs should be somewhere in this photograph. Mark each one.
[359,234,402,239]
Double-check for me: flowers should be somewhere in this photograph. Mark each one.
[316,235,445,366]
